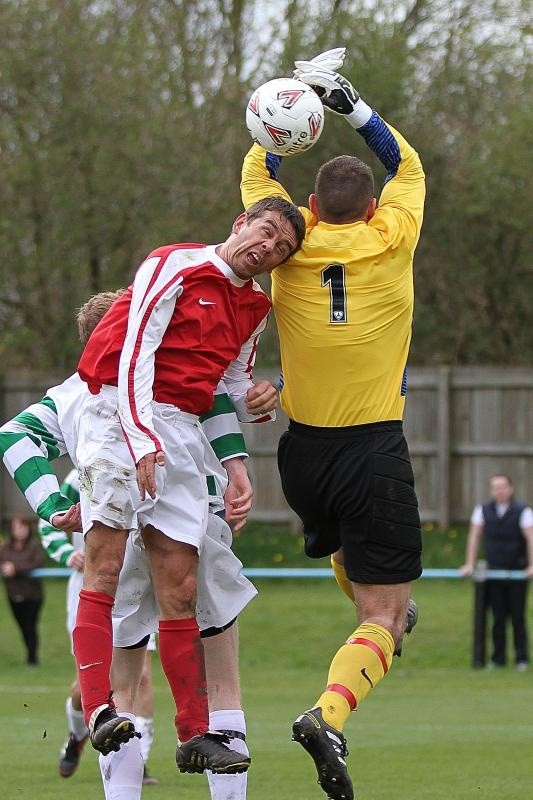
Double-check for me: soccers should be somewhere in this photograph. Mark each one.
[245,77,325,159]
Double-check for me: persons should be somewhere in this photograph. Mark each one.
[36,465,159,788]
[0,290,254,799]
[240,46,426,800]
[459,472,533,670]
[72,197,307,776]
[2,513,45,665]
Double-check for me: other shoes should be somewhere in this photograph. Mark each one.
[488,659,529,671]
[27,654,39,665]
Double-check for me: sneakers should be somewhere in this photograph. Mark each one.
[143,767,158,784]
[290,706,354,800]
[87,705,141,756]
[59,731,89,778]
[176,729,251,775]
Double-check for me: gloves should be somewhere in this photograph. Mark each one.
[293,60,372,129]
[291,48,347,81]
